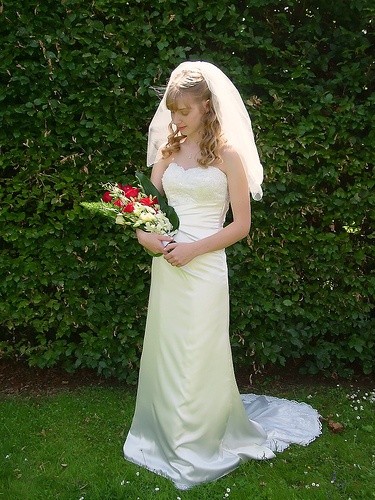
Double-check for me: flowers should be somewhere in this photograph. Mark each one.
[77,172,192,281]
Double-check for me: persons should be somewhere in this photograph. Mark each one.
[123,61,323,491]
